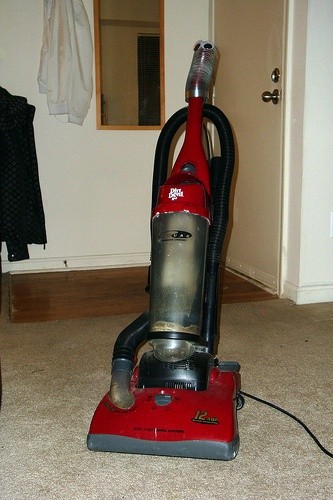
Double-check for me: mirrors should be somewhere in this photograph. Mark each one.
[92,0,167,132]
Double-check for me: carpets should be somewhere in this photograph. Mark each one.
[0,299,333,500]
